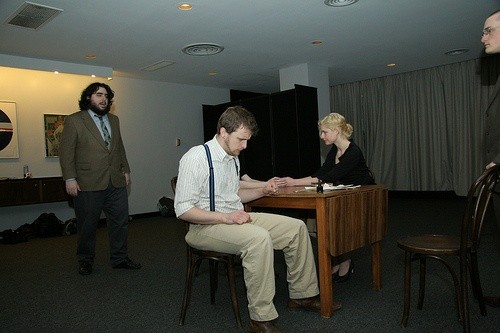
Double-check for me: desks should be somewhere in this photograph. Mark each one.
[243,183,390,319]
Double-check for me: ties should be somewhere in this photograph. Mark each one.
[94,114,112,149]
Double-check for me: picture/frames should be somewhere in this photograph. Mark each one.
[42,113,68,159]
[0,100,20,159]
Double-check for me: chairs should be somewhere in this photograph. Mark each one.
[171,175,247,329]
[396,162,500,333]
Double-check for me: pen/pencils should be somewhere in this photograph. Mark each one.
[331,188,353,191]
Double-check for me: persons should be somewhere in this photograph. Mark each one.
[239,170,286,189]
[174,104,342,333]
[271,113,378,281]
[58,82,142,275]
[479,8,500,229]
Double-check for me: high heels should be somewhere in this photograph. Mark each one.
[331,259,356,284]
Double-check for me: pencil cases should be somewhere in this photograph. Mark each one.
[311,183,333,187]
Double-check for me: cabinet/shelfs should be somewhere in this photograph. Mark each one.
[1,177,70,207]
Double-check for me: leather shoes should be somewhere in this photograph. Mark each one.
[111,258,142,271]
[247,318,286,333]
[78,260,93,276]
[288,295,343,311]
